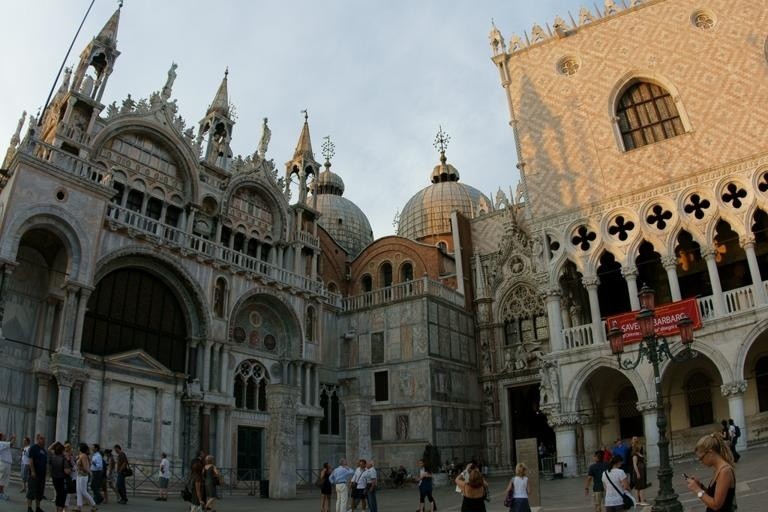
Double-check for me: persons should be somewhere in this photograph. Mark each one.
[0,431,128,512]
[189,462,205,512]
[201,454,220,512]
[154,452,170,500]
[718,419,734,462]
[538,441,546,458]
[506,463,531,512]
[319,456,491,512]
[584,436,650,512]
[196,449,206,509]
[685,432,736,512]
[728,419,743,462]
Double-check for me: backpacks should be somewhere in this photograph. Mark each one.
[735,426,740,437]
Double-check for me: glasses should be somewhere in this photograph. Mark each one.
[698,450,709,462]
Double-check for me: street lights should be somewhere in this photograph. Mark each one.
[604,281,701,512]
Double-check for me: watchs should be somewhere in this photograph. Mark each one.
[697,490,705,499]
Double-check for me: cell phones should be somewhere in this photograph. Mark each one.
[682,473,689,480]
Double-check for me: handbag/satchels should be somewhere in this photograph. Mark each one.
[622,492,637,512]
[315,479,326,486]
[124,469,133,477]
[351,482,358,489]
[504,483,515,507]
